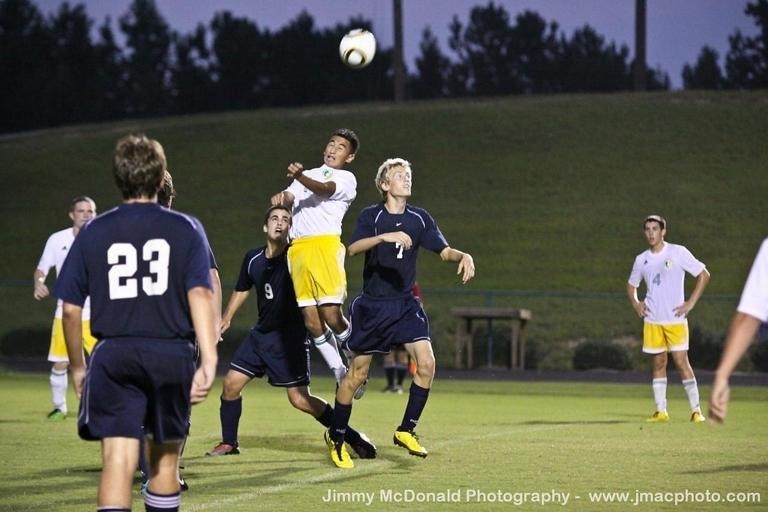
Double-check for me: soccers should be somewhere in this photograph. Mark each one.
[338,27,377,71]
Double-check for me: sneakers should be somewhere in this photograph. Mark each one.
[48,408,67,424]
[394,425,428,458]
[646,411,670,422]
[692,411,705,422]
[352,379,368,400]
[324,428,352,468]
[385,384,404,394]
[350,430,378,460]
[207,440,239,458]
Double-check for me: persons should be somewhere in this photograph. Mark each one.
[204,205,377,459]
[324,157,475,470]
[707,237,767,425]
[380,281,424,395]
[54,135,218,512]
[135,172,222,493]
[33,196,97,420]
[270,128,369,400]
[627,215,711,424]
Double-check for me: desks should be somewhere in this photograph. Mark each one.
[451,307,531,370]
